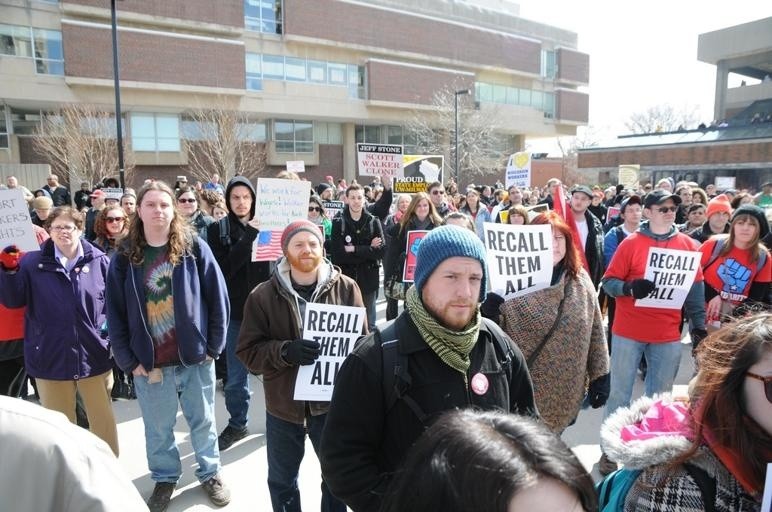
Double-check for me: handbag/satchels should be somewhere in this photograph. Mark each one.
[111,379,136,399]
[385,275,410,300]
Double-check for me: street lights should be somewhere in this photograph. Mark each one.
[455,89,471,187]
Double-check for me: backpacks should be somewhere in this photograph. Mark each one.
[594,462,717,512]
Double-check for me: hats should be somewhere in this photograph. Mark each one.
[33,196,53,210]
[621,189,681,213]
[318,184,329,195]
[89,190,120,201]
[281,220,323,254]
[176,176,188,182]
[706,194,769,238]
[572,185,592,198]
[414,225,487,304]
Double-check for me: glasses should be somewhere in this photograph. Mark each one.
[49,226,77,233]
[104,217,122,222]
[309,207,319,211]
[745,372,772,403]
[178,199,195,203]
[432,190,444,195]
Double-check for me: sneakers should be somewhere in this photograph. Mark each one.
[147,482,176,512]
[218,425,248,450]
[203,477,230,506]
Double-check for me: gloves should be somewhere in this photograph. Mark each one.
[623,279,654,299]
[287,340,319,365]
[692,328,708,349]
[588,372,610,408]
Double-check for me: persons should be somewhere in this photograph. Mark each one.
[741,80,746,87]
[719,119,729,128]
[708,121,718,128]
[678,124,686,131]
[698,123,706,129]
[761,74,772,84]
[656,125,664,132]
[750,112,772,124]
